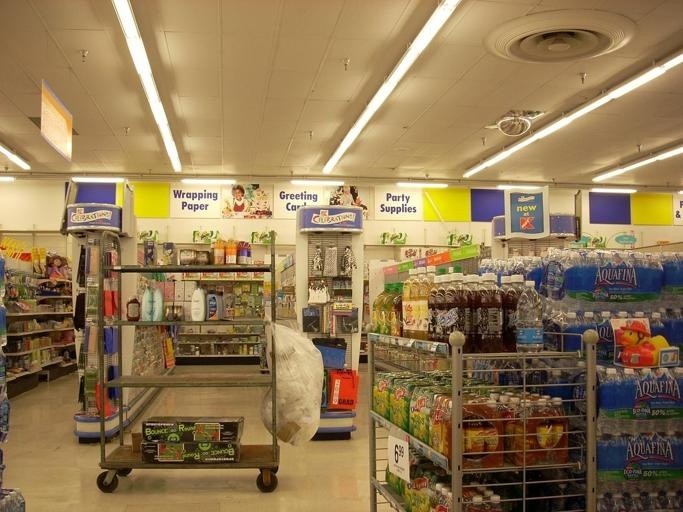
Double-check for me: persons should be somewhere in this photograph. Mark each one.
[231,185,248,213]
[340,186,359,206]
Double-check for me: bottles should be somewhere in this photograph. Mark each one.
[371,249,683,510]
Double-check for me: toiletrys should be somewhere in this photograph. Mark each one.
[191,287,206,321]
[206,288,218,322]
[141,282,152,322]
[153,286,164,322]
[216,291,224,320]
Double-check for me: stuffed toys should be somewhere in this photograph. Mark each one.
[617,320,671,367]
[48,255,73,281]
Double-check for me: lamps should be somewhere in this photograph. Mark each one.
[0,138,34,173]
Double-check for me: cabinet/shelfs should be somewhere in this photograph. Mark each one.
[128,236,174,410]
[362,241,682,512]
[274,253,298,328]
[1,253,76,386]
[161,241,270,358]
